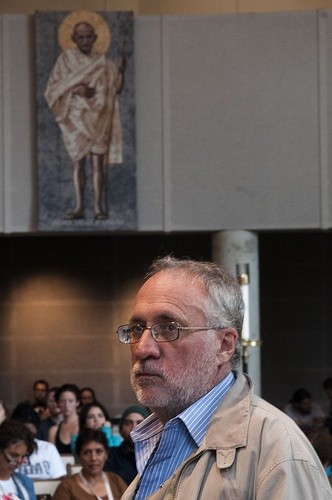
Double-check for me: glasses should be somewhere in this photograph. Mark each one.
[117,324,229,343]
[0,450,31,468]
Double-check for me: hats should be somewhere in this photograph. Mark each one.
[118,403,150,433]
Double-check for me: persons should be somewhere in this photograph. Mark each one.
[282,376,332,487]
[119,256,332,500]
[0,379,151,500]
